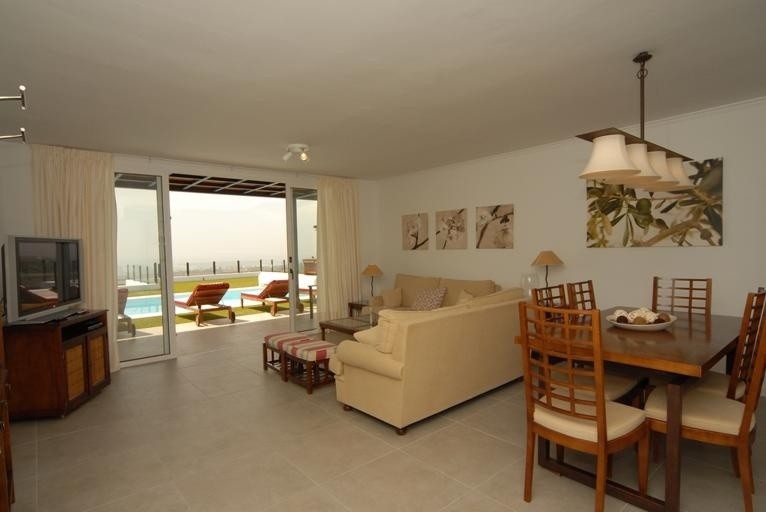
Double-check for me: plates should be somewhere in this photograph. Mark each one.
[605,314,677,331]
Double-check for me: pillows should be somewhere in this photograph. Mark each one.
[471,288,523,306]
[455,288,472,305]
[381,288,402,307]
[412,288,446,311]
[377,311,421,353]
[353,325,377,344]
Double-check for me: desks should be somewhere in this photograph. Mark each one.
[3,308,111,420]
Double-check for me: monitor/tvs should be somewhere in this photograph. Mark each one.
[0,235,85,326]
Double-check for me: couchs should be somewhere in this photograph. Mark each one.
[329,287,535,436]
[360,274,501,316]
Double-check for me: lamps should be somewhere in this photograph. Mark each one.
[360,264,384,296]
[281,143,311,167]
[531,250,564,288]
[574,50,697,192]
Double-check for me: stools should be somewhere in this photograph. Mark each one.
[261,331,315,383]
[282,339,338,395]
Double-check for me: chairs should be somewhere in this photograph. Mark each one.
[518,300,650,512]
[682,287,766,478]
[652,276,712,315]
[174,282,235,326]
[118,287,136,337]
[567,278,596,319]
[642,303,766,512]
[531,284,649,462]
[240,279,304,317]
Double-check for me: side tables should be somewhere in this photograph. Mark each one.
[348,302,368,317]
